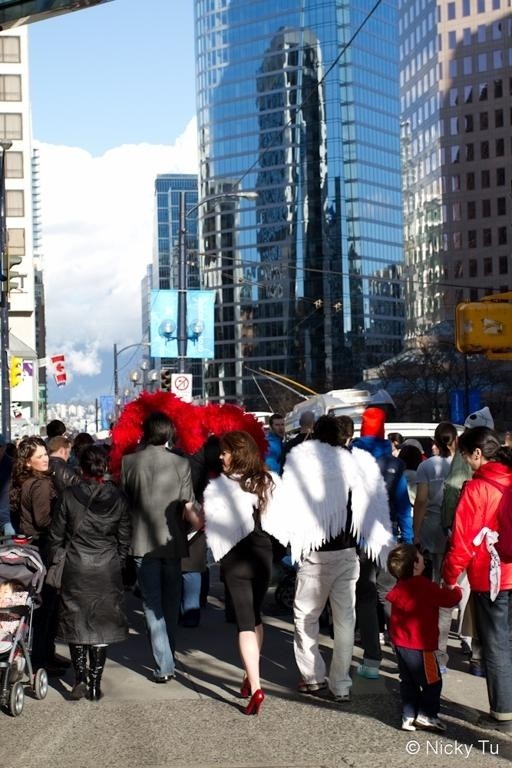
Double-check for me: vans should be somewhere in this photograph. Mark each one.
[237,385,476,475]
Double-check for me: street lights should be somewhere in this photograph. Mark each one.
[0,137,14,445]
[112,342,155,414]
[162,183,265,410]
[126,356,160,395]
[105,408,123,425]
[114,386,141,415]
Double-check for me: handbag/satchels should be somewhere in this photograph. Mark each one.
[46,548,68,589]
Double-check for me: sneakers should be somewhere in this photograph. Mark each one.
[298,682,327,692]
[357,664,379,680]
[401,715,448,733]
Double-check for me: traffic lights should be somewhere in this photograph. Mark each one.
[0,252,22,293]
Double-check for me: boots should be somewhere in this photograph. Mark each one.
[67,644,88,698]
[88,645,107,701]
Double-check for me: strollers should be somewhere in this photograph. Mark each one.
[0,527,57,715]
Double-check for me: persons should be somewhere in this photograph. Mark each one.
[1,406,512,729]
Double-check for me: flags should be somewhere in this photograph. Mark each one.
[52,355,69,385]
[9,354,22,387]
[22,360,32,377]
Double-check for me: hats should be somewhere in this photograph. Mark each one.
[464,405,495,429]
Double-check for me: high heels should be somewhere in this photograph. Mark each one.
[240,679,265,715]
